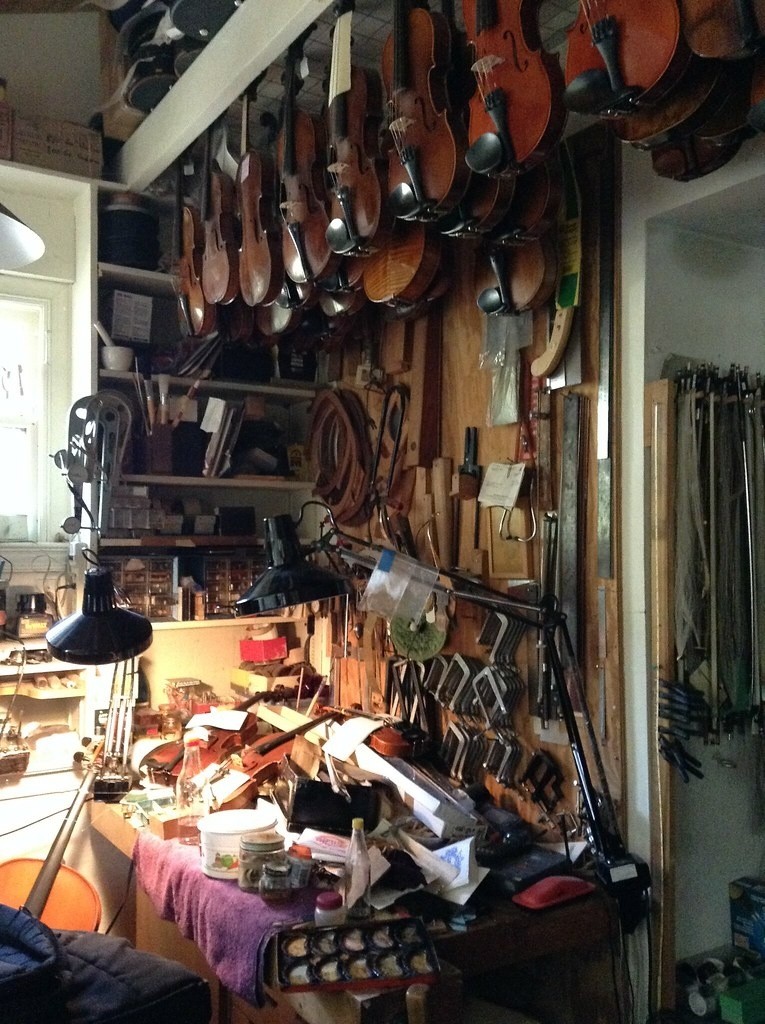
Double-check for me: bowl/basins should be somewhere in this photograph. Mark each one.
[197,808,278,880]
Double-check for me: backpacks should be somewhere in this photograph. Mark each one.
[1,905,72,1024]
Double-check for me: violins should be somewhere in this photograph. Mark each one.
[139,685,287,788]
[202,703,360,813]
[173,0,765,344]
[313,699,430,755]
[0,738,116,933]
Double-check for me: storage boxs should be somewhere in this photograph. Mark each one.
[728,877,765,959]
[105,484,215,536]
[718,977,765,1024]
[0,9,147,178]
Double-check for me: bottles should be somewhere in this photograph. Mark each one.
[135,701,183,740]
[314,892,345,927]
[237,833,285,893]
[344,818,372,918]
[176,737,211,846]
[283,843,312,889]
[258,860,292,902]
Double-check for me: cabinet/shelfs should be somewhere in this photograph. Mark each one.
[70,189,332,628]
[0,542,86,769]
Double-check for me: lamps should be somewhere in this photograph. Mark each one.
[45,567,154,668]
[236,502,347,615]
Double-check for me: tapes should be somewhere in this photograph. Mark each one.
[245,623,279,641]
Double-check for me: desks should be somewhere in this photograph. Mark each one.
[90,790,624,1024]
